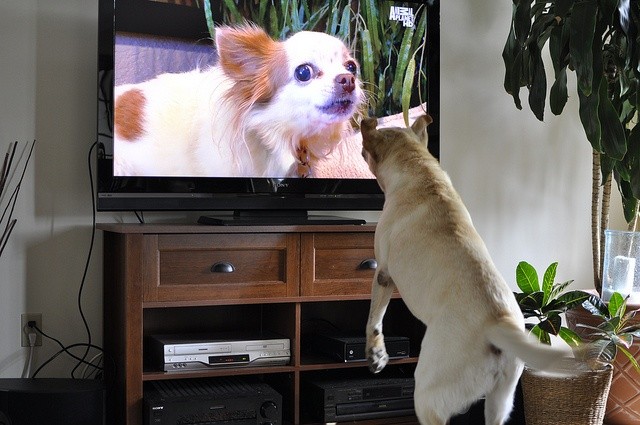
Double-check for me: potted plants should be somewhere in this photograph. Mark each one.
[497,0,640,424]
[510,261,639,423]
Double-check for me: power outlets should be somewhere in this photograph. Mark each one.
[20,315,44,349]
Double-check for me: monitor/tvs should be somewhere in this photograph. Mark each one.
[95,0,440,226]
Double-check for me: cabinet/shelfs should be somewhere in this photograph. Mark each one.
[95,222,419,425]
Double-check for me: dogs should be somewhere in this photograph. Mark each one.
[114,16,374,178]
[361,115,597,425]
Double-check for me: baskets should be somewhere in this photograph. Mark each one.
[520,361,614,425]
